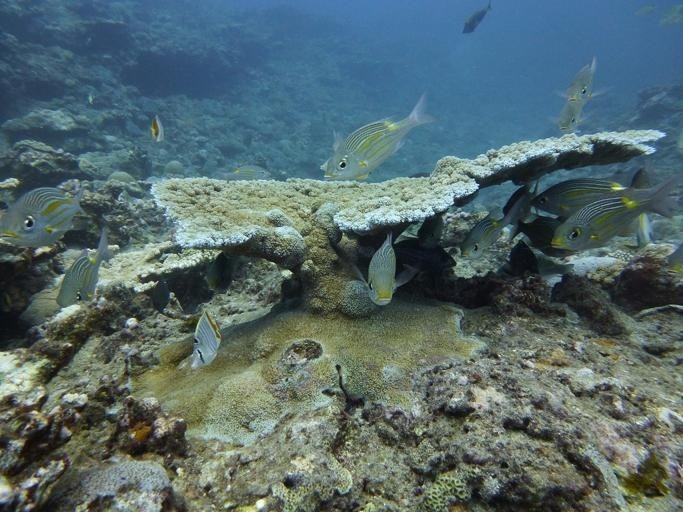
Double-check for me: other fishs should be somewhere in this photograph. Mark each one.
[459,163,673,285]
[553,55,603,132]
[352,207,457,308]
[150,114,168,147]
[1,179,121,309]
[460,1,495,34]
[321,92,435,181]
[186,309,223,367]
[222,164,272,182]
